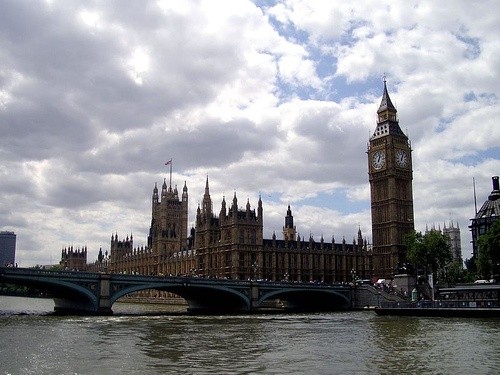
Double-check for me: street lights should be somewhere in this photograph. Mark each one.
[190,267,196,278]
[350,268,356,286]
[102,250,111,274]
[252,261,259,282]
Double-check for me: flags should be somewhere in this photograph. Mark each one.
[165,160,171,165]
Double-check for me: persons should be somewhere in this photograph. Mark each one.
[152,271,230,280]
[7,263,14,267]
[32,264,79,276]
[372,280,500,302]
[98,270,141,275]
[247,278,356,286]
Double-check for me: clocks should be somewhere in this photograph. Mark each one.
[394,149,409,168]
[371,150,385,169]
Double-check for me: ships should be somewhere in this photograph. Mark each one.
[374,287,500,317]
